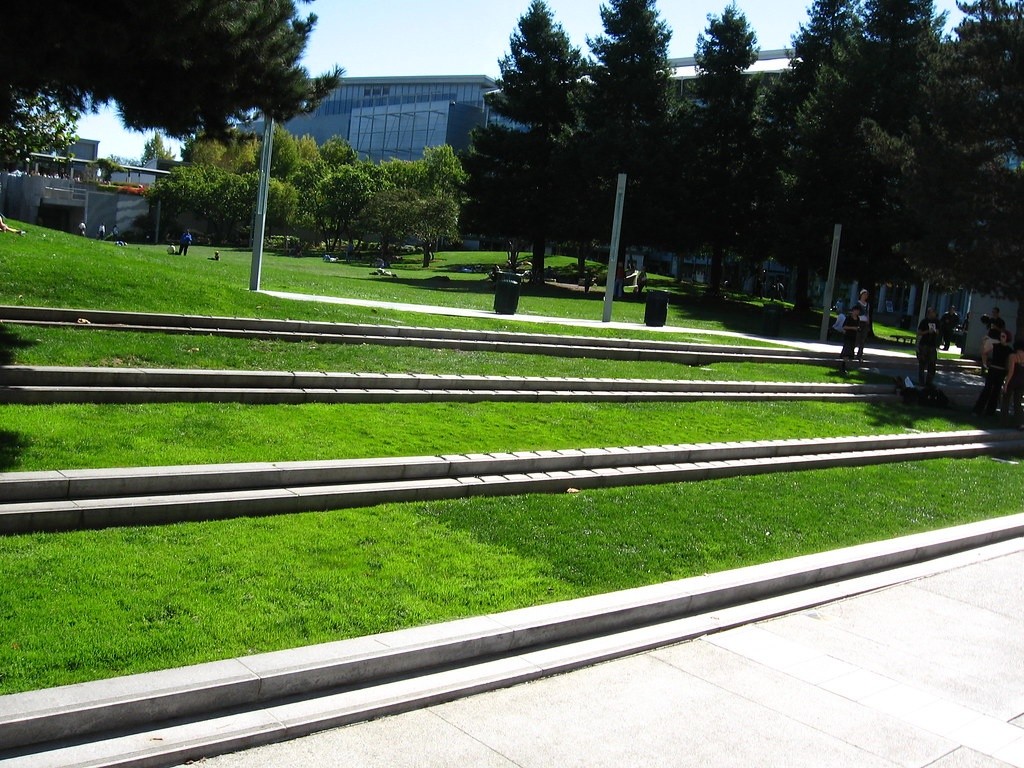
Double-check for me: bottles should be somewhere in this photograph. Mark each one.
[982,367,990,375]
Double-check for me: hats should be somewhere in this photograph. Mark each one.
[860,289,868,294]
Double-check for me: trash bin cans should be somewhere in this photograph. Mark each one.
[762,303,784,336]
[493,270,523,315]
[643,288,672,327]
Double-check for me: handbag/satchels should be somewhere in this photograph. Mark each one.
[858,315,868,321]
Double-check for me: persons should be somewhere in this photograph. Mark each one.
[614,262,648,302]
[839,303,861,359]
[323,254,330,261]
[369,245,555,281]
[3,164,112,185]
[166,242,176,254]
[346,241,355,263]
[1001,332,1024,431]
[972,329,1013,419]
[856,288,870,359]
[78,219,121,241]
[178,228,192,256]
[916,307,943,387]
[0,213,26,237]
[939,304,960,351]
[984,307,1005,335]
[962,311,971,335]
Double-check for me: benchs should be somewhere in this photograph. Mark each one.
[890,335,917,344]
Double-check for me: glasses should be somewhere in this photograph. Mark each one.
[1002,335,1005,338]
